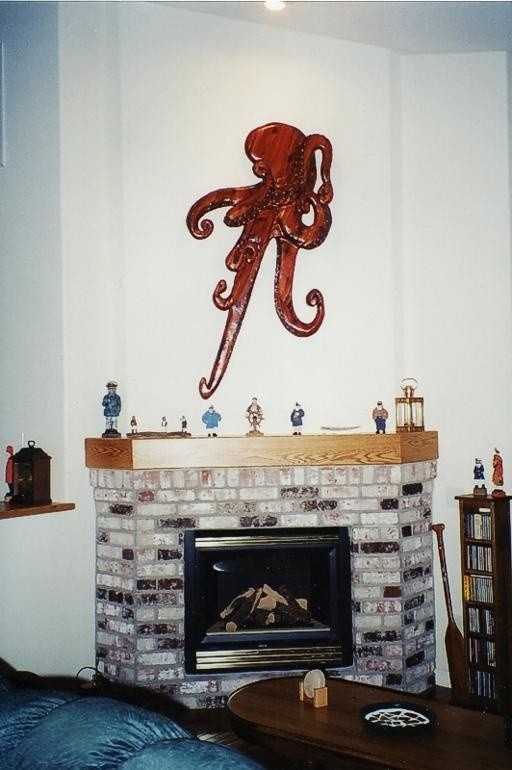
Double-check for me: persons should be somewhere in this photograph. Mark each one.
[473,458,485,490]
[131,416,138,434]
[246,398,262,427]
[372,401,388,434]
[161,417,168,436]
[202,405,222,437]
[290,402,304,435]
[492,448,504,486]
[102,381,122,435]
[180,416,187,433]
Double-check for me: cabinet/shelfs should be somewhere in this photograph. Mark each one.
[457,492,512,712]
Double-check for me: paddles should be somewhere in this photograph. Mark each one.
[435,523,467,697]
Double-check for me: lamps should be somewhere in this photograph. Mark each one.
[6,440,54,509]
[396,373,424,433]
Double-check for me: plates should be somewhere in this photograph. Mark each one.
[360,700,439,732]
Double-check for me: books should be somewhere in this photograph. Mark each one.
[461,506,500,702]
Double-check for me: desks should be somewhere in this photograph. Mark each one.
[227,668,512,770]
